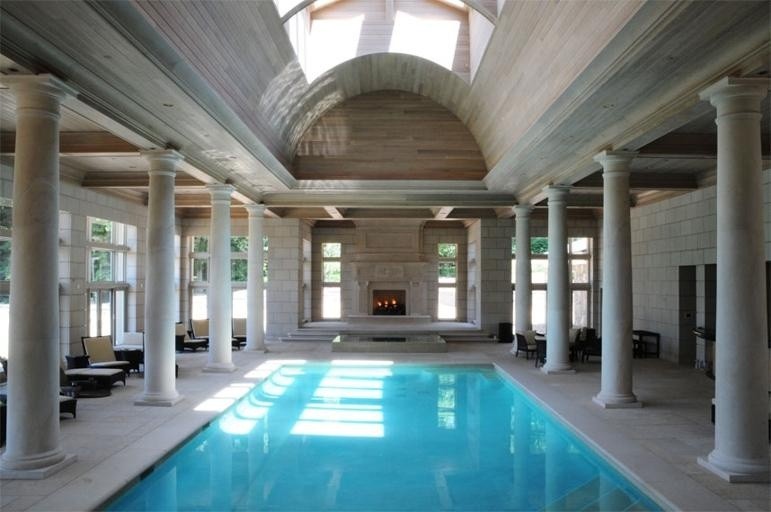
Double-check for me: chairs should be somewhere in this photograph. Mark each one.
[516,325,604,368]
[1,319,247,435]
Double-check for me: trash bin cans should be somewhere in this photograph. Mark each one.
[498,323,512,343]
[176,321,186,351]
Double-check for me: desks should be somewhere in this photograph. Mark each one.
[633,330,660,359]
